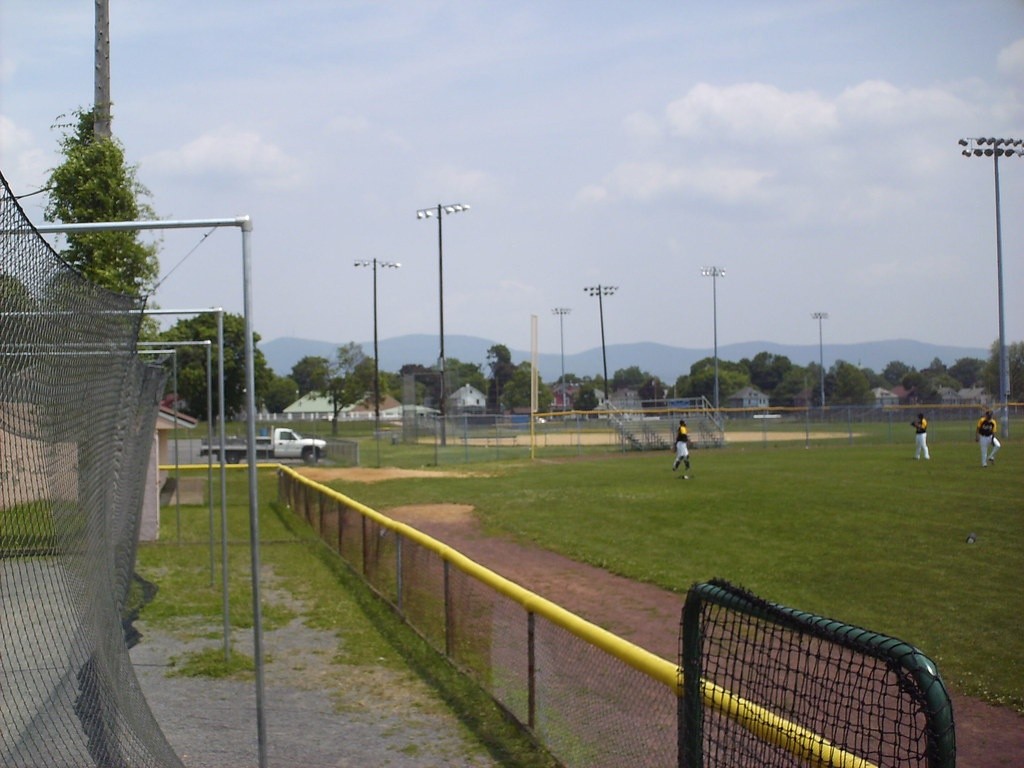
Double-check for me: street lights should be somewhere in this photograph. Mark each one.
[552,305,573,424]
[959,133,1024,438]
[583,284,620,405]
[353,257,403,435]
[811,313,829,417]
[699,266,726,417]
[416,200,470,448]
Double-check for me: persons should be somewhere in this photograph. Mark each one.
[673,420,690,470]
[976,411,1001,467]
[911,413,930,460]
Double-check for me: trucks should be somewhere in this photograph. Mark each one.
[199,428,328,464]
